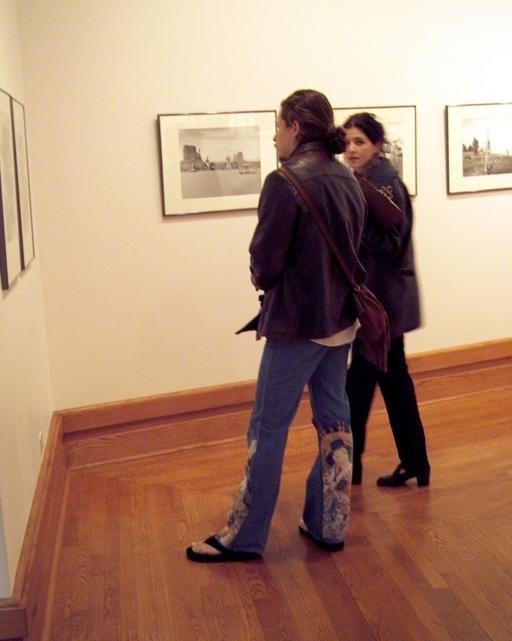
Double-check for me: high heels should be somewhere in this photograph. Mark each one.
[376,463,430,488]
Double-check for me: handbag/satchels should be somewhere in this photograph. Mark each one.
[356,285,391,360]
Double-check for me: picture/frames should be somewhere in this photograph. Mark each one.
[156,110,277,217]
[445,102,512,195]
[331,105,417,197]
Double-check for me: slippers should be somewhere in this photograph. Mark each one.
[185,535,263,564]
[298,519,344,552]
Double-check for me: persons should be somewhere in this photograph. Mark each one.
[337,111,433,490]
[184,87,371,564]
